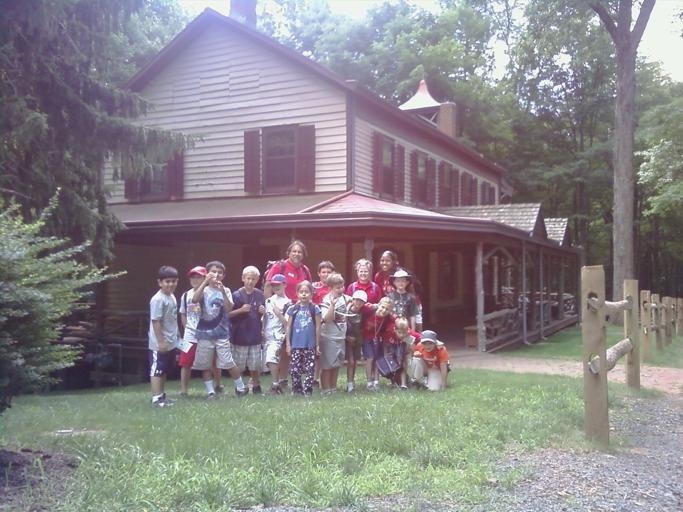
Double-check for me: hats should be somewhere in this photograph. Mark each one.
[420,330,436,343]
[269,274,285,284]
[188,266,207,276]
[353,290,367,302]
[390,270,410,277]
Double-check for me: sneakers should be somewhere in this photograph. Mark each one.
[154,381,407,405]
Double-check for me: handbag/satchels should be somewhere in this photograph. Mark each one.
[376,353,400,378]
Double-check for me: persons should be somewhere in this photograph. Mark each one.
[346,256,383,304]
[373,316,446,392]
[359,296,393,391]
[341,289,367,391]
[410,329,449,393]
[284,280,321,397]
[310,259,336,305]
[380,268,419,390]
[262,239,312,390]
[374,249,422,332]
[257,273,292,397]
[177,266,225,399]
[223,265,266,398]
[190,260,246,400]
[144,265,181,408]
[318,272,354,397]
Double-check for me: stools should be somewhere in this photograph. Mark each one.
[462,325,477,350]
